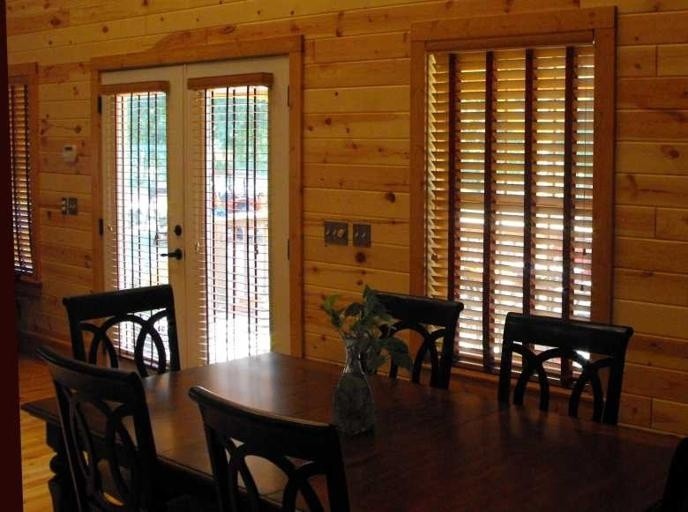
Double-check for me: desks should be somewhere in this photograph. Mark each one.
[19,350,681,510]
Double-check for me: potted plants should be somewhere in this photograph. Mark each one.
[323,293,412,439]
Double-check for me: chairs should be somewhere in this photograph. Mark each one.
[62,284,180,377]
[662,437,687,512]
[33,345,203,511]
[360,289,464,389]
[497,312,634,424]
[188,386,350,512]
